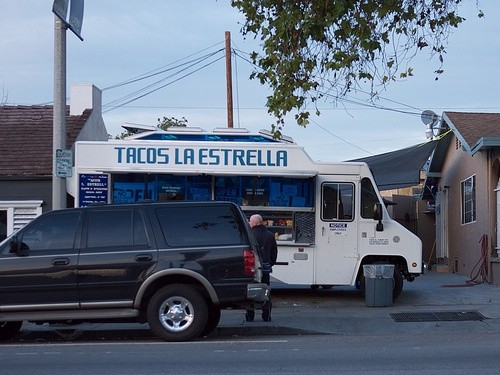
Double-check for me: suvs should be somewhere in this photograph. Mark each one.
[0,199,269,341]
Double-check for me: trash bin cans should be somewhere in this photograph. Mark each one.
[362,264,395,307]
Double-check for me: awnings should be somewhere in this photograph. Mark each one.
[341,138,436,190]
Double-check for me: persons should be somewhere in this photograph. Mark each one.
[241,215,278,323]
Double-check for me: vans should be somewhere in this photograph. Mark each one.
[74,124,427,300]
